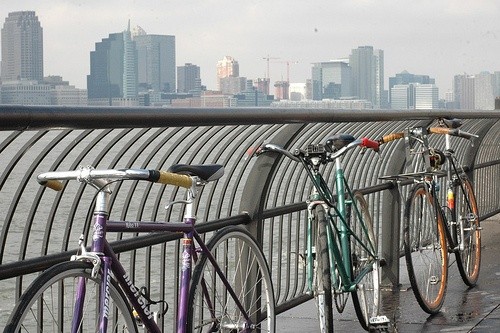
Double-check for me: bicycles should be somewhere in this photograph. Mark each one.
[4,164,277,333]
[358,117,482,314]
[249,132,391,333]
